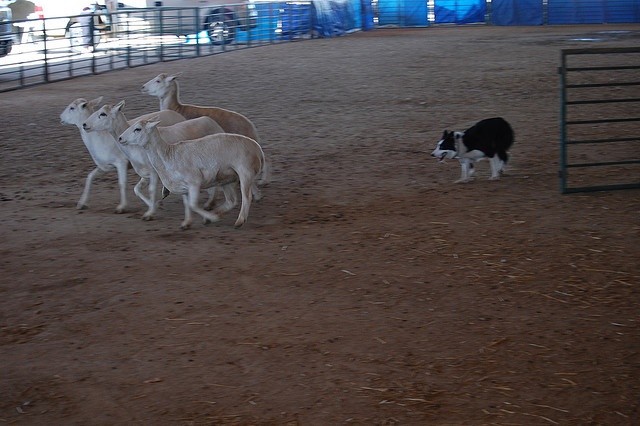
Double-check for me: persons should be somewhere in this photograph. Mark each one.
[76,7,103,52]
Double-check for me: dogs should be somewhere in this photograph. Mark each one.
[430,116,515,183]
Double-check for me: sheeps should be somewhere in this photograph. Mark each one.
[58,94,187,214]
[117,113,266,230]
[82,98,264,220]
[140,70,263,147]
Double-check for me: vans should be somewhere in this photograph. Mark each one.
[145,0,259,45]
[0,6,13,56]
